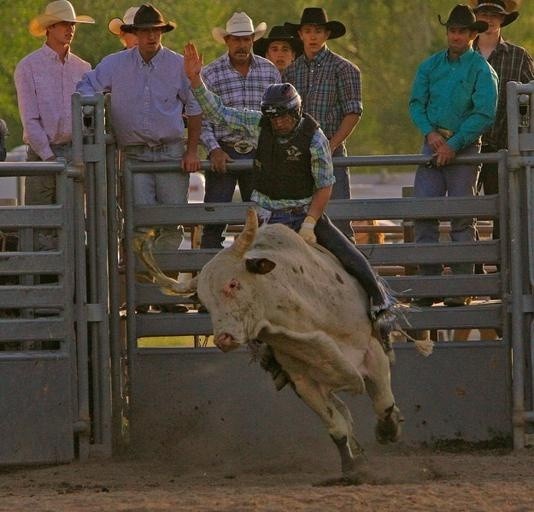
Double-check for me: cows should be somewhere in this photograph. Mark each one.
[137,203,435,485]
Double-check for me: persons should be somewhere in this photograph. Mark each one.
[253,27,302,70]
[76,4,202,315]
[280,5,363,244]
[12,1,94,284]
[406,6,498,307]
[468,0,534,301]
[184,41,397,332]
[198,12,279,314]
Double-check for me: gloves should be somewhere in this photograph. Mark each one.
[297,215,317,243]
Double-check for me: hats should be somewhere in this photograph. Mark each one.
[212,7,346,59]
[109,7,141,37]
[472,3,519,27]
[438,4,488,34]
[29,2,95,38]
[120,5,176,34]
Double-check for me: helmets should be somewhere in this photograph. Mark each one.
[261,83,304,137]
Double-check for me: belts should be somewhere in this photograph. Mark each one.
[437,126,453,138]
